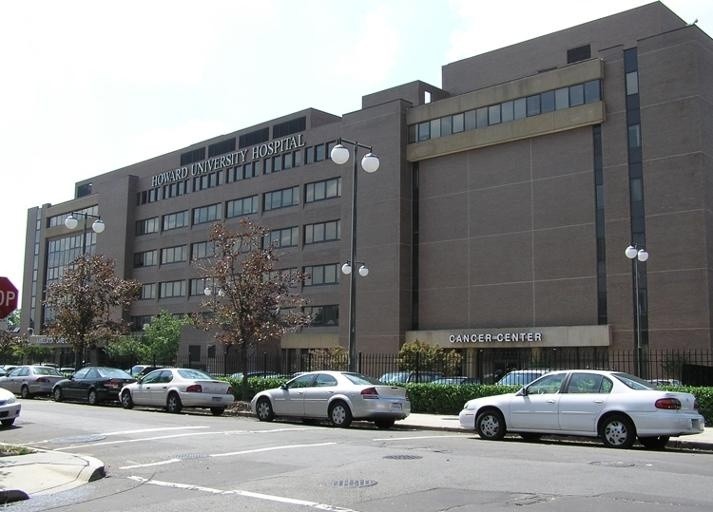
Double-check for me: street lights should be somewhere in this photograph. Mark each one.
[203,282,227,323]
[622,238,648,348]
[329,137,379,372]
[63,210,105,369]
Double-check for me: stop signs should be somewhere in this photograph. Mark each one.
[0,276,18,320]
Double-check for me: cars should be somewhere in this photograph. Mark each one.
[224,370,485,428]
[51,365,235,413]
[458,369,705,449]
[0,362,76,425]
